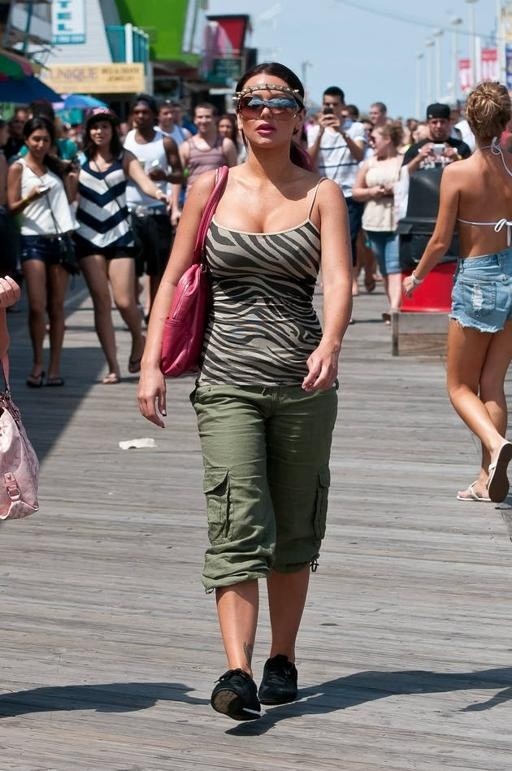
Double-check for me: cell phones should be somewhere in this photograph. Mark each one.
[37,184,51,194]
[323,107,335,117]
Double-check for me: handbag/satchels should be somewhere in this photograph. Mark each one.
[55,231,85,276]
[130,228,145,278]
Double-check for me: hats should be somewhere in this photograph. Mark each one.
[84,105,119,126]
[426,102,452,123]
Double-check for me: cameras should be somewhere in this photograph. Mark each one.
[431,143,446,156]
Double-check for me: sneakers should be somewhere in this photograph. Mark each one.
[210,666,262,721]
[257,653,299,705]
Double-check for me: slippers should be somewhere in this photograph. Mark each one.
[128,334,145,373]
[45,372,65,388]
[101,372,122,384]
[364,275,376,293]
[485,441,511,503]
[26,368,45,389]
[456,477,496,502]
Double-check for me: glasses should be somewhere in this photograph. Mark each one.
[235,95,301,121]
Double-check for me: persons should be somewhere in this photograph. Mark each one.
[135,63,354,724]
[295,83,511,502]
[0,92,247,392]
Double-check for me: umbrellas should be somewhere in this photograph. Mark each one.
[0,49,110,128]
